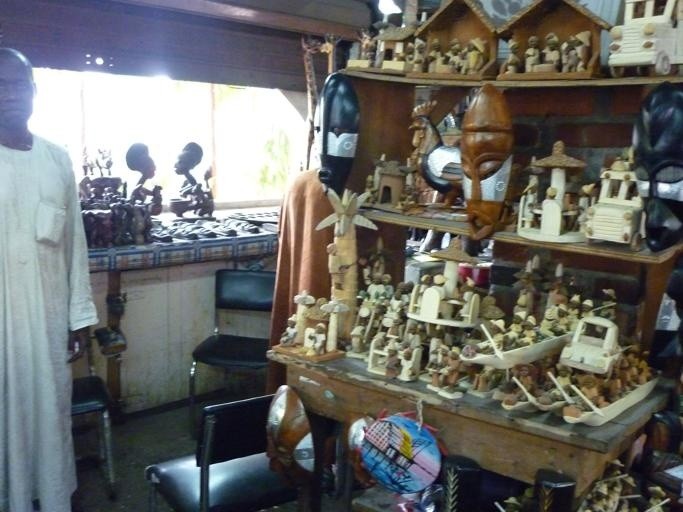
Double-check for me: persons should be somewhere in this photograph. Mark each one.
[1,46,99,511]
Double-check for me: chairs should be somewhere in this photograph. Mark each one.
[143,393,299,512]
[188,269,276,417]
[71,326,127,501]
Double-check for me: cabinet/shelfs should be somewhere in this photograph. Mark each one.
[266,69,683,511]
